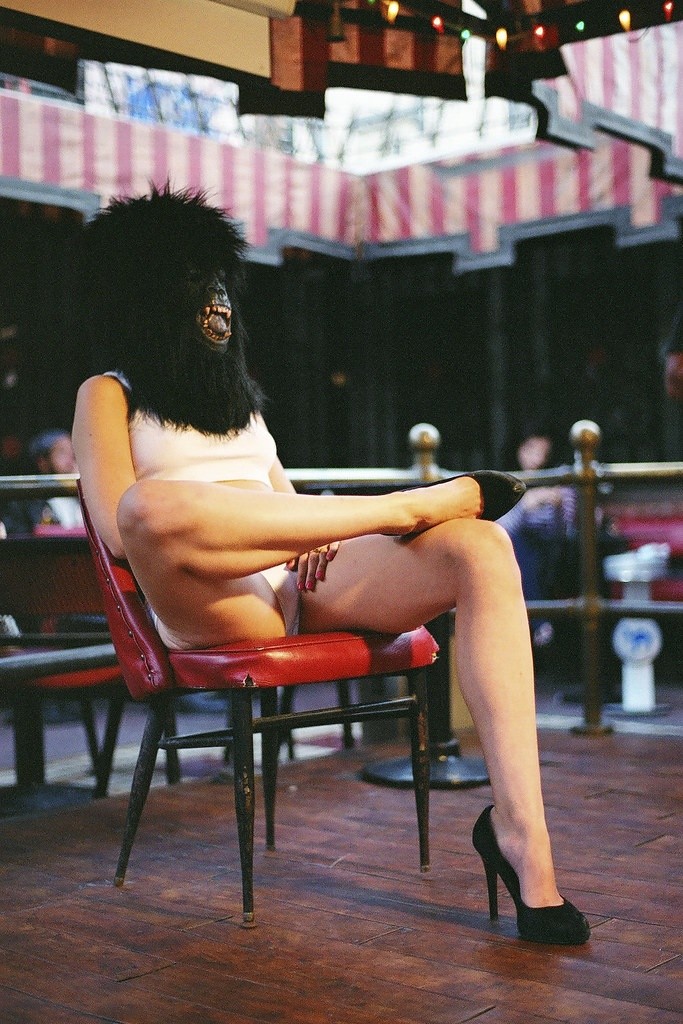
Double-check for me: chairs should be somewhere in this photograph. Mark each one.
[0,473,181,798]
[76,473,440,928]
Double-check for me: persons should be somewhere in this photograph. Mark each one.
[492,433,630,703]
[69,182,593,946]
[0,424,120,634]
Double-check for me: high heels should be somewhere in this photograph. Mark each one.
[470,804,591,944]
[379,470,526,538]
